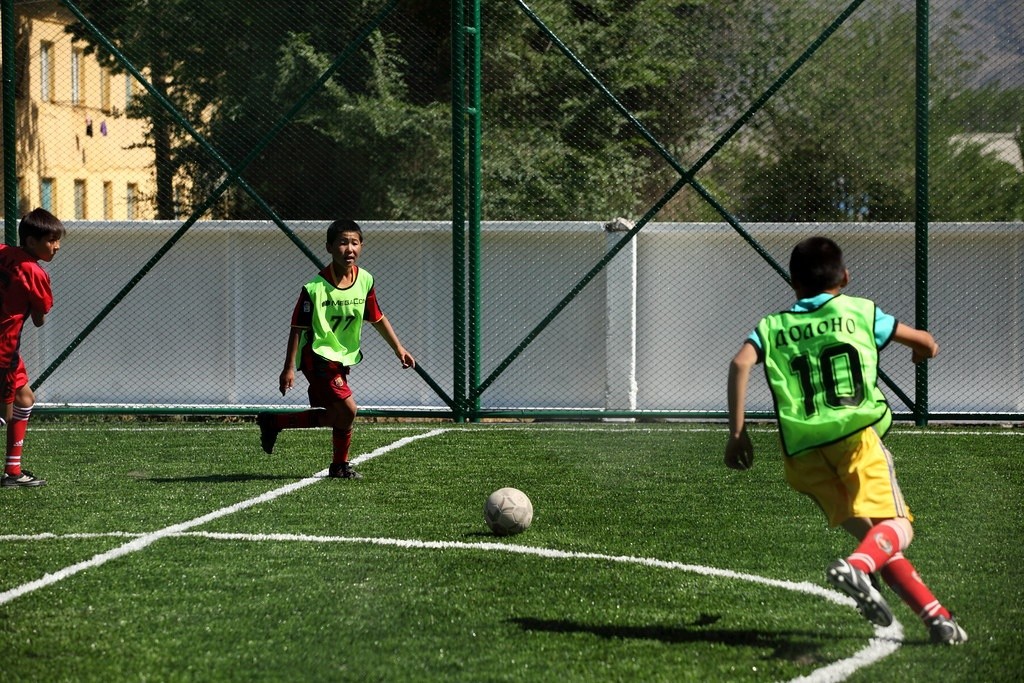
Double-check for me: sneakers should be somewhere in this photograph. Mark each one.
[1,469,47,487]
[329,462,363,479]
[929,614,968,646]
[255,411,282,454]
[826,557,892,627]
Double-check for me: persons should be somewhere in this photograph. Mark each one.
[0,207,66,488]
[254,219,415,480]
[728,237,969,648]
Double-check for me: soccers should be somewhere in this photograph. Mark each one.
[484,487,534,536]
